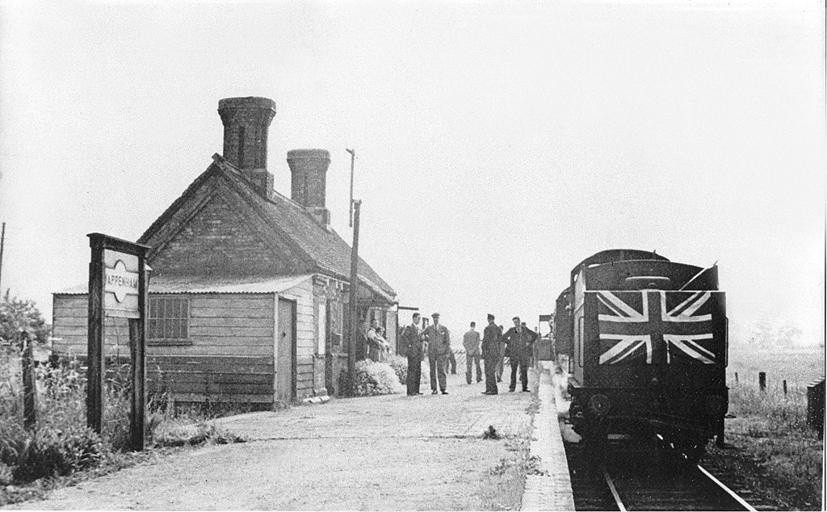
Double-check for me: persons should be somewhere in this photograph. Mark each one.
[400,312,423,396]
[365,318,381,361]
[420,311,449,396]
[460,321,482,384]
[494,324,503,381]
[499,317,538,392]
[479,313,500,395]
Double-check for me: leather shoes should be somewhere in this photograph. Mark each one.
[509,388,515,392]
[442,391,449,394]
[523,389,530,392]
[486,391,497,395]
[432,390,438,394]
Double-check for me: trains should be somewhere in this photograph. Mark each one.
[551,248,729,465]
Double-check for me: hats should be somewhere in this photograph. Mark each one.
[432,313,440,317]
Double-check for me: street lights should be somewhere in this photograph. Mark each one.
[344,149,357,230]
